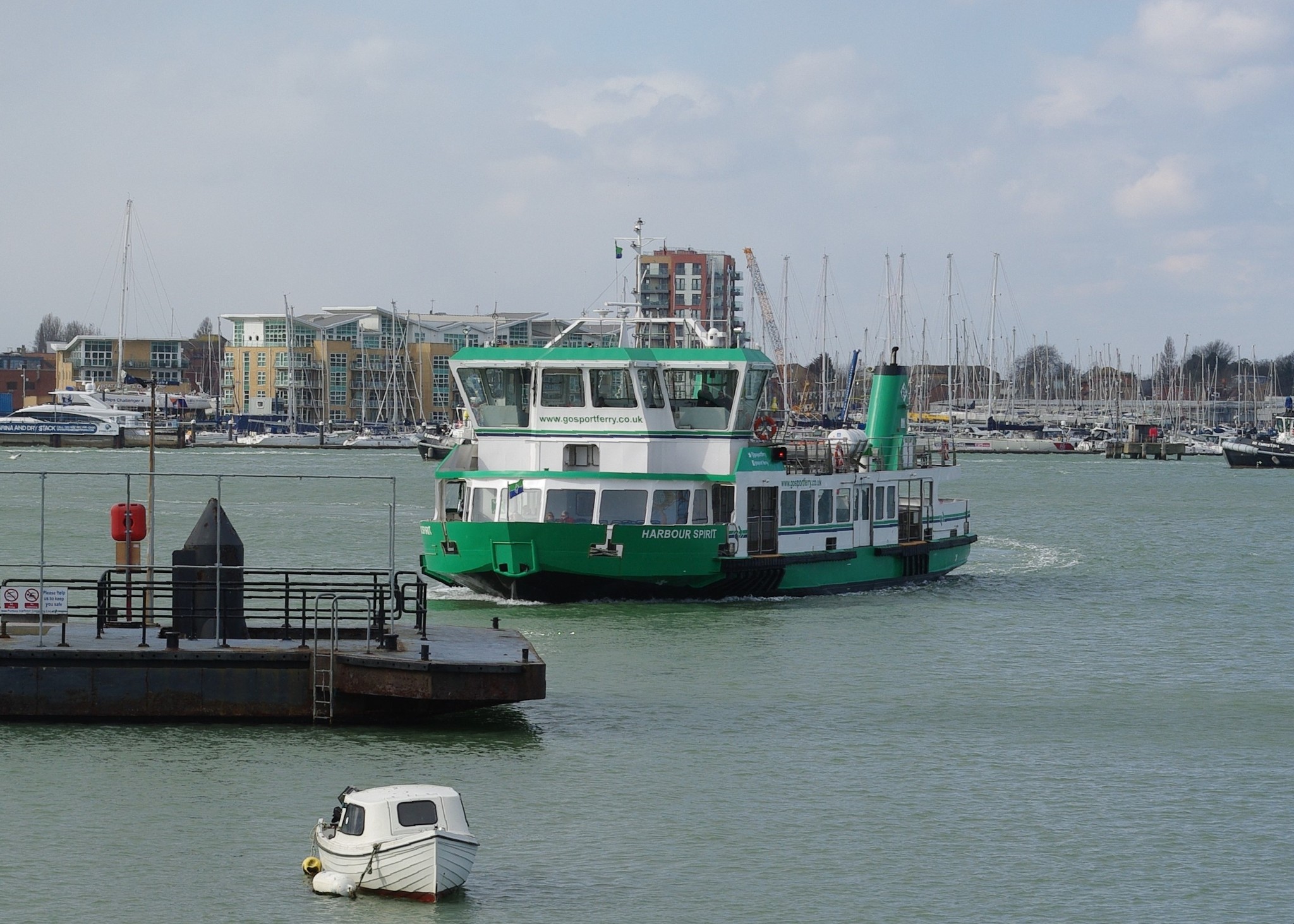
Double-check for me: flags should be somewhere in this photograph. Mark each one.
[616,246,623,258]
[508,479,524,498]
[121,369,146,388]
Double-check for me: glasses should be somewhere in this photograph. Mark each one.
[718,393,723,394]
[561,515,566,517]
[548,515,553,516]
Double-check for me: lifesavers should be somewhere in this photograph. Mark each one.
[835,445,844,466]
[942,439,949,460]
[754,415,777,440]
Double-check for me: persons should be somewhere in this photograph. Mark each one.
[546,510,574,523]
[714,390,730,410]
[696,385,715,407]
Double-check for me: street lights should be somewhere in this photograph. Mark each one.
[21,368,29,398]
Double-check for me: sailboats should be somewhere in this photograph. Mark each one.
[56,199,214,411]
[738,251,1294,470]
[180,295,429,449]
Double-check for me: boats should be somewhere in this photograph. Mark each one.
[315,782,479,903]
[7,386,179,436]
[418,216,979,602]
